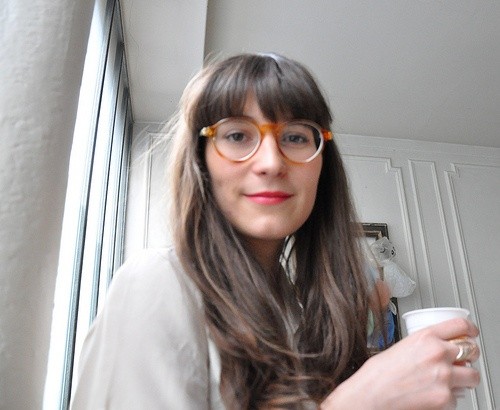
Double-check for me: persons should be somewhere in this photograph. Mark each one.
[69,52,481,410]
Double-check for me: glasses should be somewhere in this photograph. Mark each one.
[199,116,332,165]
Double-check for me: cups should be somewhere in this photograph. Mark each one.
[402,308,470,397]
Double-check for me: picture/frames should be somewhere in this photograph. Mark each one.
[350,222,400,352]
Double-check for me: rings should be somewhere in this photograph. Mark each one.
[455,340,476,360]
